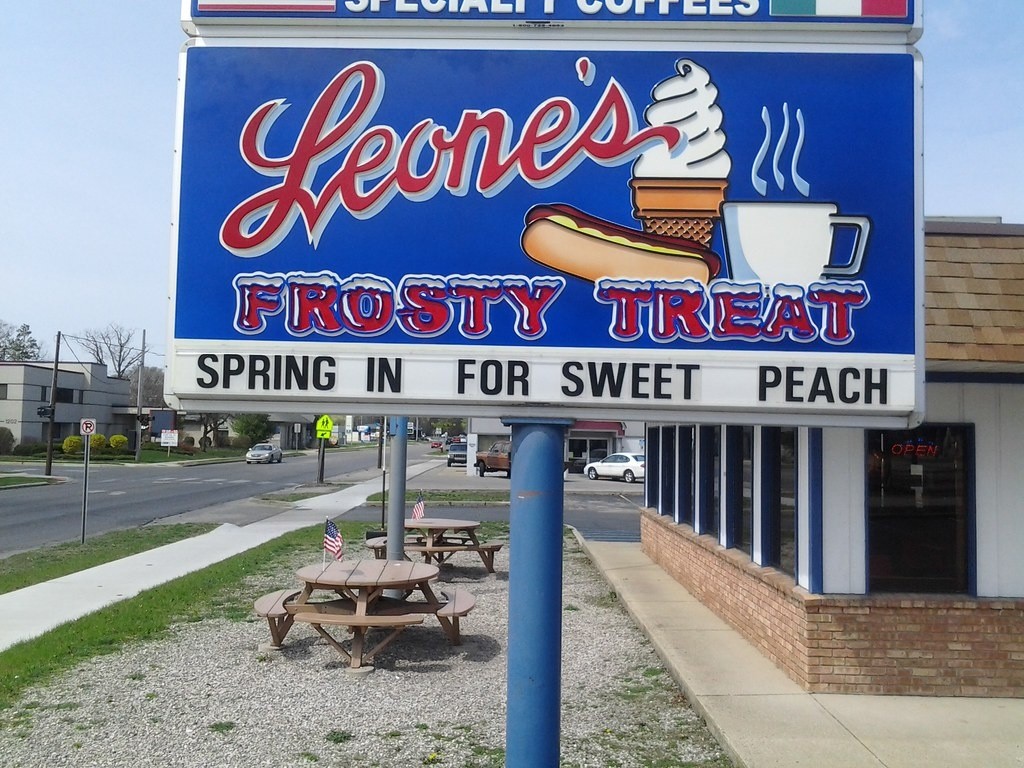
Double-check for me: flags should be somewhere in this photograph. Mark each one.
[412,491,424,520]
[324,519,344,562]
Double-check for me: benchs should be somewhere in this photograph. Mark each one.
[293,612,425,625]
[366,537,387,549]
[437,589,477,617]
[404,546,468,551]
[253,589,301,617]
[479,540,505,549]
[407,535,471,540]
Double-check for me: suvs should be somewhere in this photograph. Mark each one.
[446,443,467,467]
[473,440,512,478]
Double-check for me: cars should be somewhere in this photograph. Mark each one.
[445,434,467,445]
[441,433,445,438]
[245,444,283,465]
[431,441,443,448]
[591,448,607,463]
[584,453,645,483]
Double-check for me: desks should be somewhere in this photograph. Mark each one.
[368,518,496,585]
[254,560,474,678]
